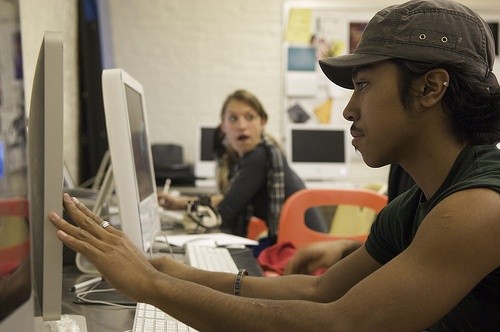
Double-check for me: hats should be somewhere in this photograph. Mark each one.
[318,0,495,90]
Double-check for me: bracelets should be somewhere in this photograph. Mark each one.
[234,268,248,296]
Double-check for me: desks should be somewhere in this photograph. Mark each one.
[0,179,386,332]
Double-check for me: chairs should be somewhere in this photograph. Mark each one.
[263,188,387,276]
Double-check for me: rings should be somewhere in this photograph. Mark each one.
[99,220,109,229]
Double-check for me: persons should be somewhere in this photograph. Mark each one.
[158,89,327,257]
[49,1,500,332]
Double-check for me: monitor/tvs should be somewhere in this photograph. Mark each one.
[101,68,162,254]
[286,123,350,181]
[194,123,224,188]
[24,29,87,332]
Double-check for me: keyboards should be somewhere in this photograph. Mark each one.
[131,302,199,332]
[183,243,240,277]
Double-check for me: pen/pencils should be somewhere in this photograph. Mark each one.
[159,178,172,205]
[70,277,103,291]
